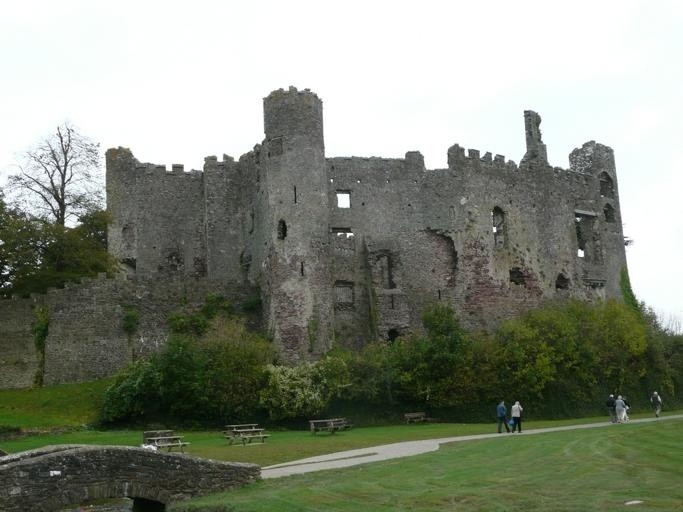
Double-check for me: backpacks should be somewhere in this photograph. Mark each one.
[652,395,658,405]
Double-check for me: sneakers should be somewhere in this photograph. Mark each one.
[518,431,523,433]
[507,430,512,432]
[511,431,515,433]
[498,432,504,433]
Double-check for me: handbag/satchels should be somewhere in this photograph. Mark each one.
[509,417,516,425]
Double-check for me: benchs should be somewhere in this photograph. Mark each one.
[221,424,271,447]
[308,416,353,432]
[143,429,191,455]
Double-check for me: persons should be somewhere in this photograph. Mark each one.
[621,396,630,423]
[605,393,617,424]
[494,399,509,434]
[613,395,626,424]
[508,400,524,433]
[649,390,663,418]
